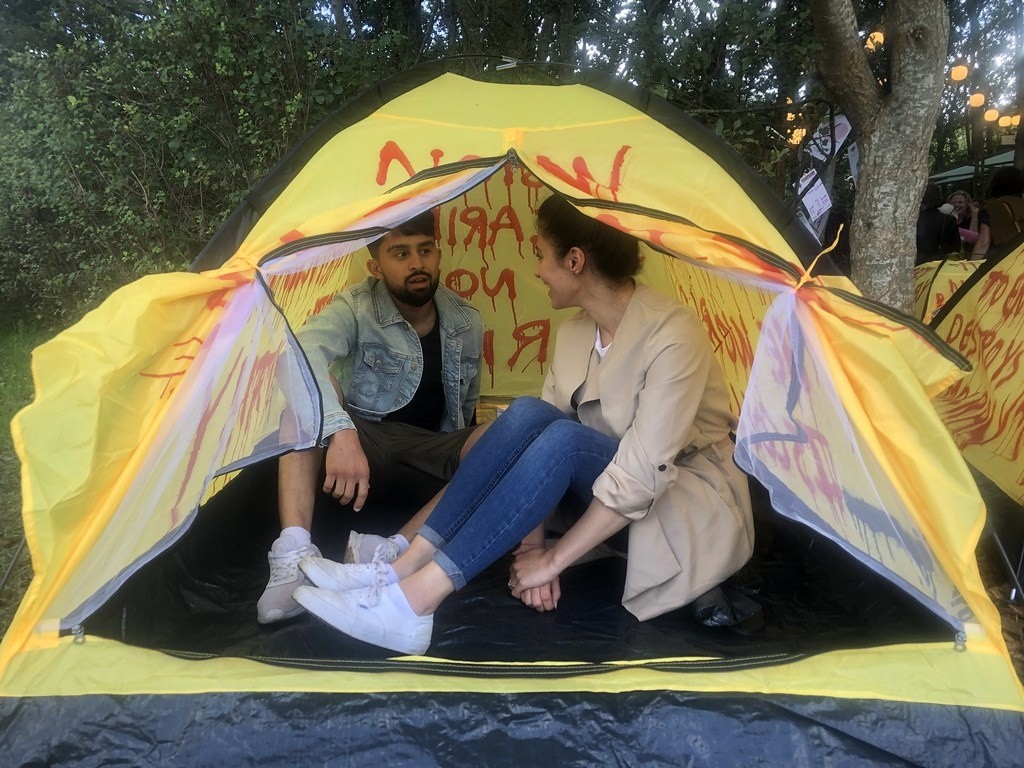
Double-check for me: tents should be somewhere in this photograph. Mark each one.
[919,239,1024,543]
[0,57,1022,768]
[912,254,988,329]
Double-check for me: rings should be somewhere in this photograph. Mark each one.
[507,579,517,588]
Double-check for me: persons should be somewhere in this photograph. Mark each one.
[916,184,962,263]
[947,191,992,261]
[292,192,756,658]
[257,208,503,625]
[937,203,980,253]
[972,168,1024,259]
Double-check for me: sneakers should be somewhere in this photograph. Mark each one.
[341,529,399,565]
[256,535,323,625]
[292,582,434,657]
[299,554,391,589]
